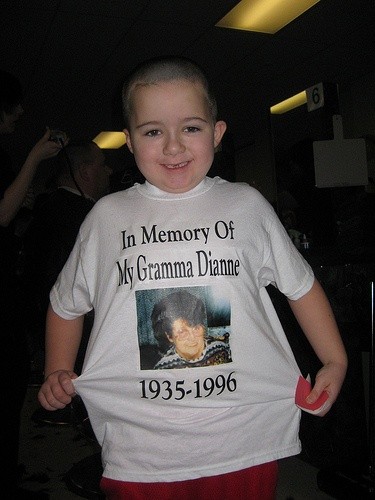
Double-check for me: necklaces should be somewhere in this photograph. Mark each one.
[62,184,77,190]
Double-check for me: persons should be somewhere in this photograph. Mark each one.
[36,56,348,500]
[0,72,115,500]
